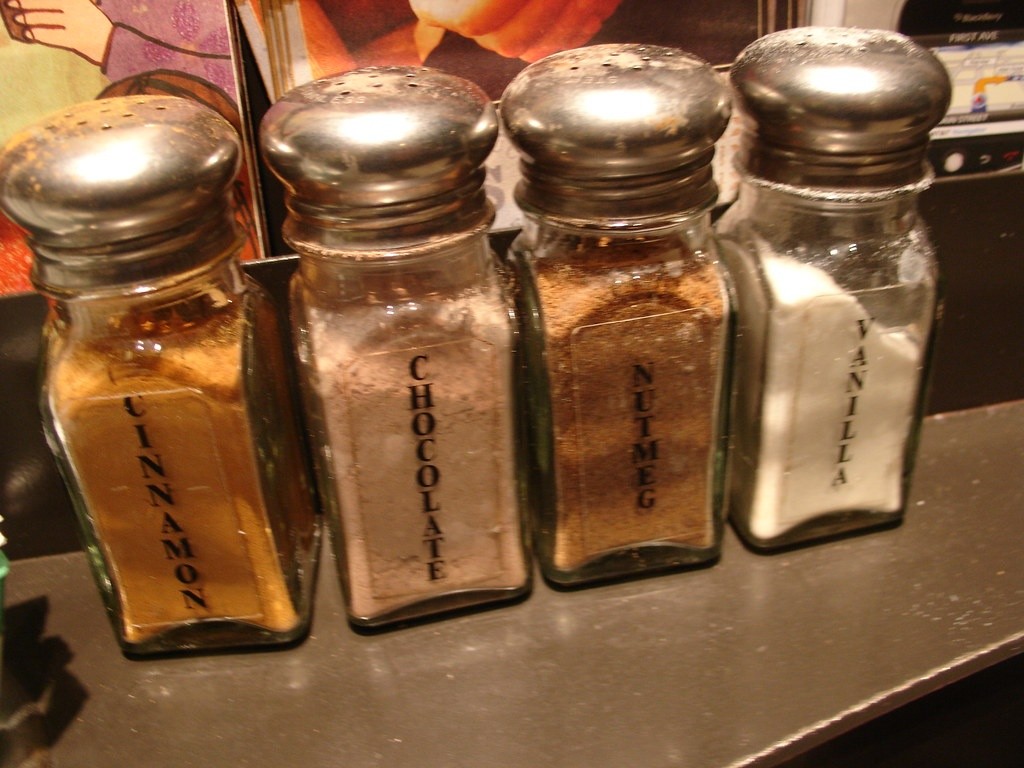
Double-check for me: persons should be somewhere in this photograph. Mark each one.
[408,0,623,93]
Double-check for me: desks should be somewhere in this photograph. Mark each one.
[0,395,1024,768]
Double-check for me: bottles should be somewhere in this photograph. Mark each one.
[257,65,536,632]
[490,44,740,591]
[0,97,325,662]
[713,25,950,558]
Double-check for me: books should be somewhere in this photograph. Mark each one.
[236,0,349,106]
